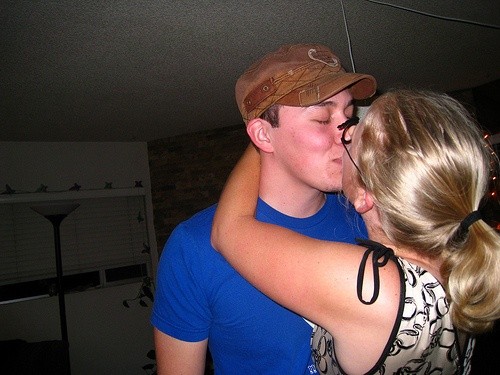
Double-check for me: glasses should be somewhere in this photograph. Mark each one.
[337,116,362,174]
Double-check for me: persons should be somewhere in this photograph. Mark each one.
[211,89,500,374]
[151,44,378,375]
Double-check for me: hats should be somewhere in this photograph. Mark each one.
[234,42,377,127]
